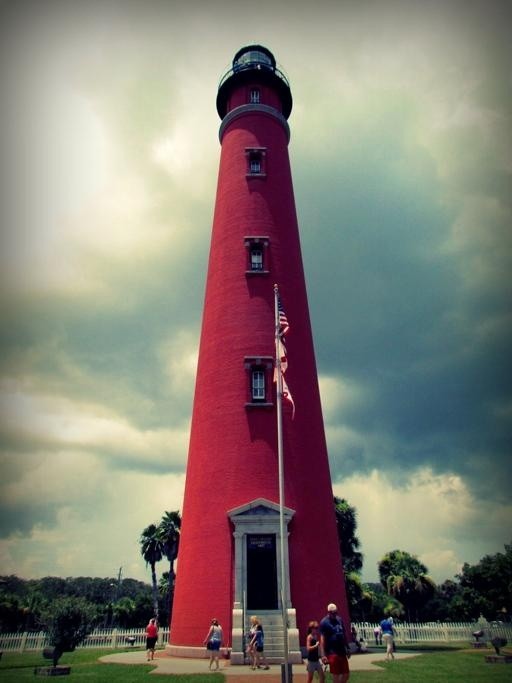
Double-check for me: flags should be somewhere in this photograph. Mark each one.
[273,291,295,420]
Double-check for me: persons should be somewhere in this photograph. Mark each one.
[305,620,326,683]
[145,619,158,661]
[249,625,270,670]
[379,615,399,660]
[351,626,365,651]
[248,616,260,669]
[203,618,223,672]
[319,603,352,683]
[374,626,383,645]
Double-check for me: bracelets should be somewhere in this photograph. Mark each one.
[347,647,351,650]
[320,652,326,657]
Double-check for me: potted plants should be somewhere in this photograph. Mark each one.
[33,600,104,676]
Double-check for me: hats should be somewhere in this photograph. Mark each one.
[328,603,337,614]
